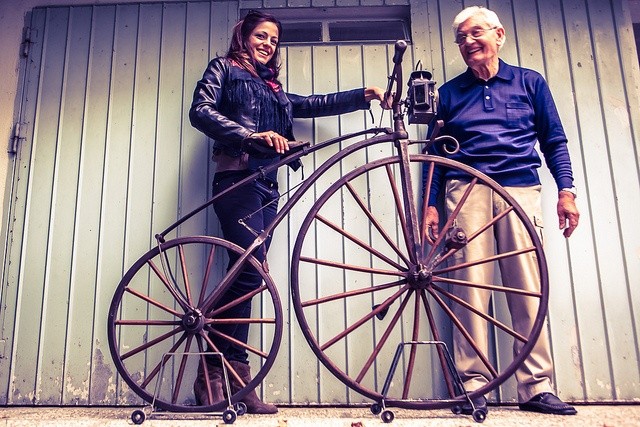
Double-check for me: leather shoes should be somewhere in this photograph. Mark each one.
[519,392,577,414]
[461,391,488,414]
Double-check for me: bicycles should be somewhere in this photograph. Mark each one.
[106,39,551,423]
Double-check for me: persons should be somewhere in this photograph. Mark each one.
[189,10,395,413]
[419,5,580,415]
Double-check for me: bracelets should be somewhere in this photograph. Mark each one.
[561,187,579,198]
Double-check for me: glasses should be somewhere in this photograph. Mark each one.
[454,26,497,44]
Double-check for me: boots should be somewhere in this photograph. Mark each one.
[193,358,227,412]
[222,360,278,413]
